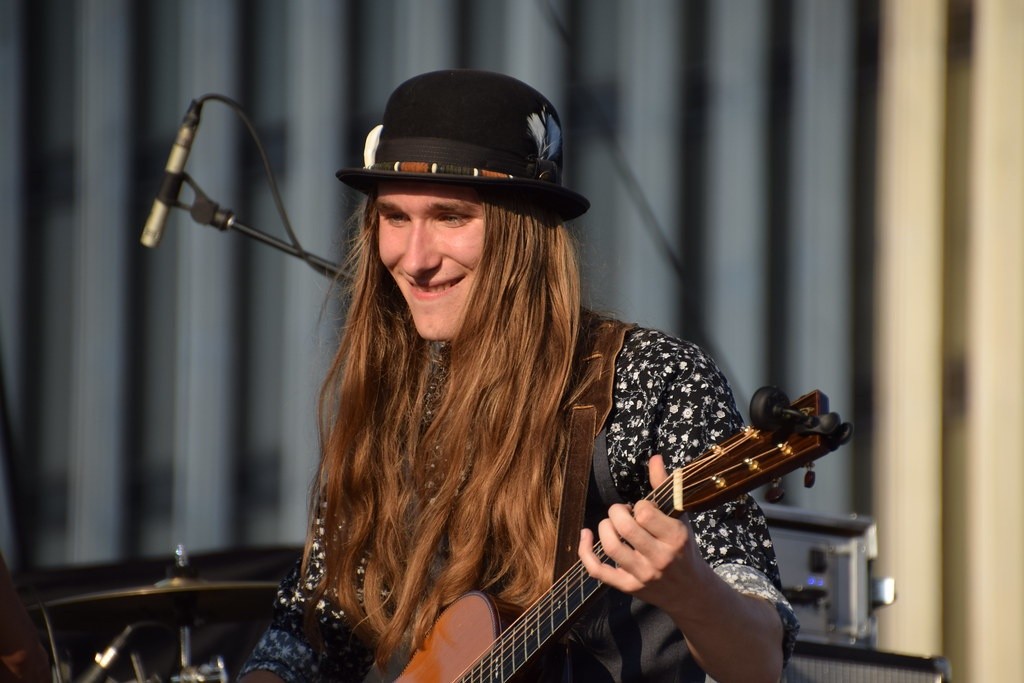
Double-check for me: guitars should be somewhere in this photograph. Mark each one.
[362,388,853,683]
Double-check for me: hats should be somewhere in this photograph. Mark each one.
[335,69,592,222]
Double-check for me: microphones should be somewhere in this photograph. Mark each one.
[81,626,134,683]
[141,100,204,248]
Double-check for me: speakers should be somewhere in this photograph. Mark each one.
[704,640,953,683]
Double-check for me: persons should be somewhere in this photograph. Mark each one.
[235,69,799,683]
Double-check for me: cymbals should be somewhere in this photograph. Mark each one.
[23,580,281,632]
